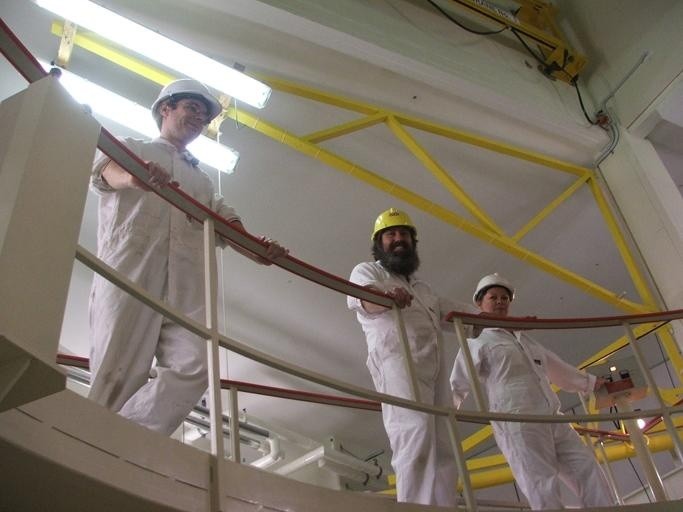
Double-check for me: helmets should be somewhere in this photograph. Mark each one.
[370,207,418,242]
[472,272,516,309]
[150,79,222,134]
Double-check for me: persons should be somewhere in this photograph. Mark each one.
[448,273,615,509]
[346,208,537,508]
[84,79,290,438]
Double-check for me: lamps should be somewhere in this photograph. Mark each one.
[34,0,271,110]
[35,58,240,173]
